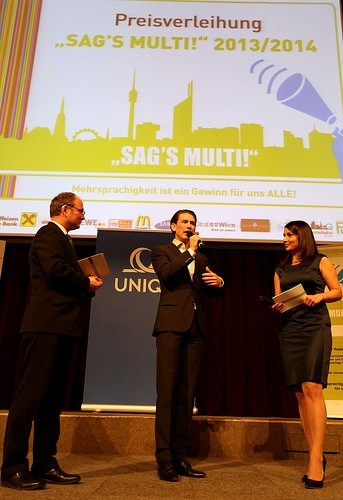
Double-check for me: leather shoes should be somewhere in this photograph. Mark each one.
[1,470,47,491]
[158,466,179,482]
[174,459,206,477]
[30,467,81,484]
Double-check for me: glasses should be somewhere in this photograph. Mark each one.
[65,202,86,215]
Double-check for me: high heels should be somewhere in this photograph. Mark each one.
[301,454,327,489]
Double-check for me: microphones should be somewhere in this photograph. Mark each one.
[187,231,205,249]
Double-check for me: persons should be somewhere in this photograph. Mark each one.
[0,192,104,490]
[150,209,227,482]
[271,220,343,490]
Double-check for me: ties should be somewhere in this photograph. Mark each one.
[66,235,74,249]
